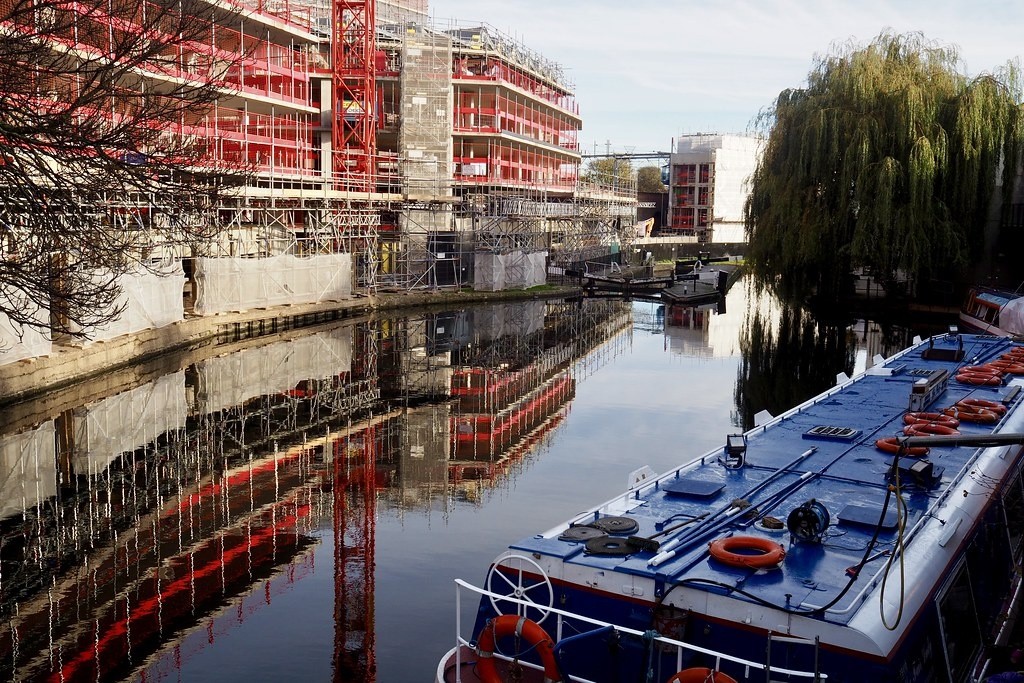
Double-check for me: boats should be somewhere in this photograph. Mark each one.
[437,323,1024,683]
[953,272,1024,337]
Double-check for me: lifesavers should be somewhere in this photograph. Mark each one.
[475,614,561,683]
[955,346,1024,386]
[667,666,739,683]
[877,398,1008,455]
[708,535,786,567]
